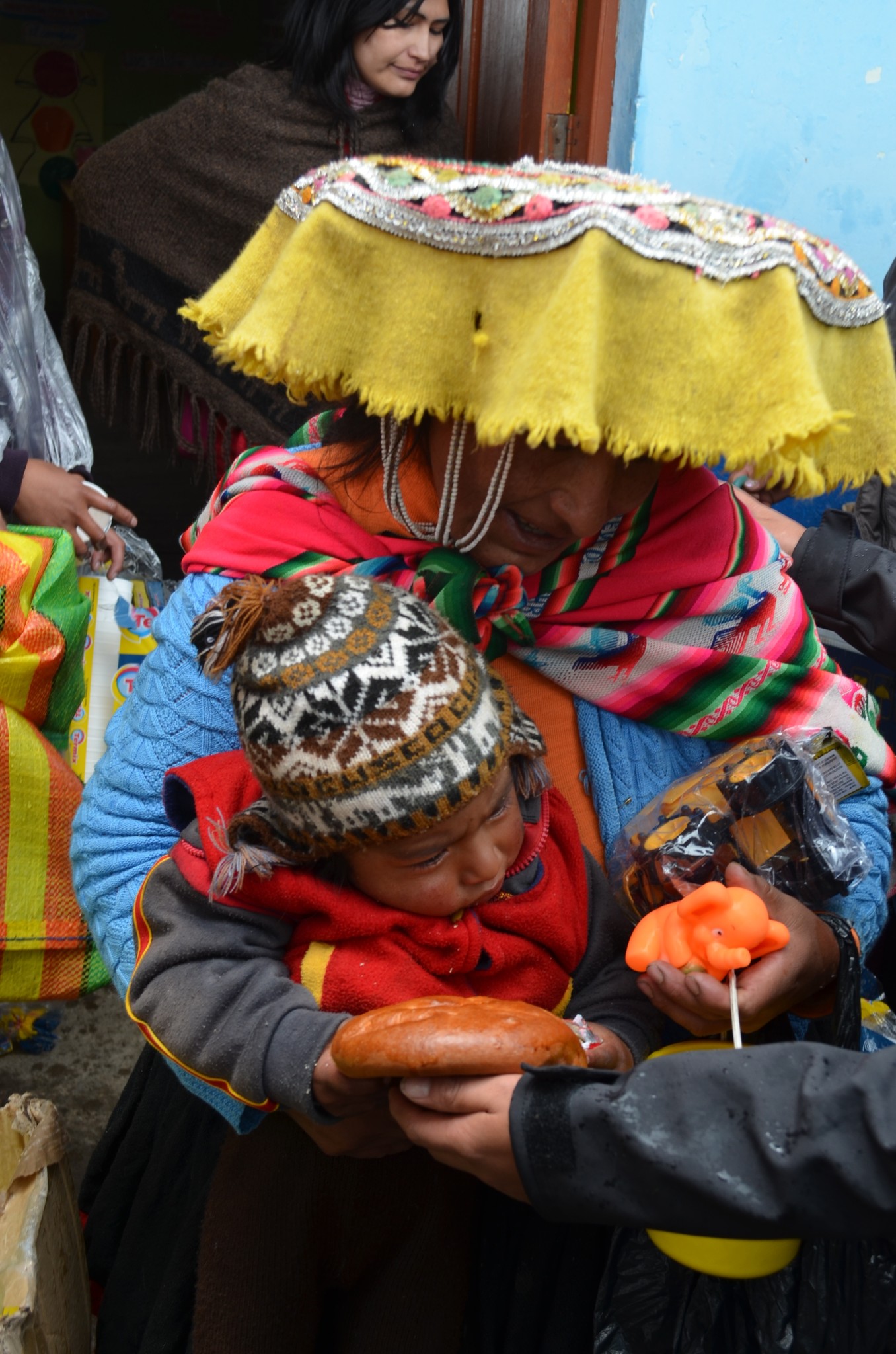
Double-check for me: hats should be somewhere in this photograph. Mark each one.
[178,156,896,498]
[195,572,553,906]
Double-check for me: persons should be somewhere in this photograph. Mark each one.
[0,0,896,1354]
[126,565,896,1354]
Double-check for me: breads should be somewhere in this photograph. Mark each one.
[331,996,588,1079]
